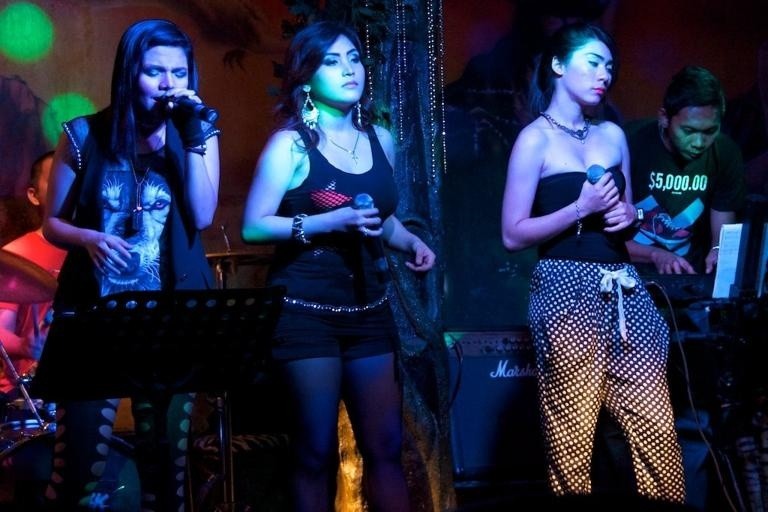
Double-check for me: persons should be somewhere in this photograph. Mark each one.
[502,27,689,509]
[620,64,748,274]
[240,19,435,512]
[0,147,110,393]
[44,19,222,512]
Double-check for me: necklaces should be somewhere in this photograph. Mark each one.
[322,133,362,167]
[539,111,590,144]
[130,131,168,216]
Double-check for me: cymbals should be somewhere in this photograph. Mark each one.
[206,251,268,269]
[1,246,58,305]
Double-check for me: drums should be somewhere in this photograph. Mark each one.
[0,391,56,428]
[0,419,144,512]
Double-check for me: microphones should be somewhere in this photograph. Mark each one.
[587,164,633,241]
[354,193,388,273]
[160,94,218,123]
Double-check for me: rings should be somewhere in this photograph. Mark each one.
[364,228,368,237]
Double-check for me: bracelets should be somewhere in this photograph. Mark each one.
[573,201,583,238]
[711,244,719,250]
[290,214,312,245]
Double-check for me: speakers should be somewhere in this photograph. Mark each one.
[443,330,544,486]
